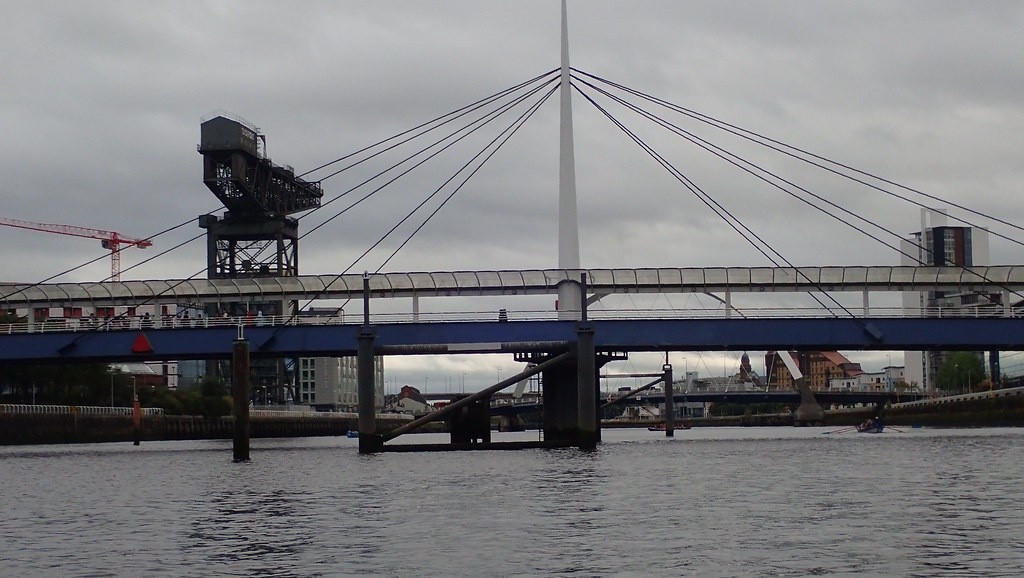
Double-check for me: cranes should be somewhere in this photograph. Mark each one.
[1,217,153,282]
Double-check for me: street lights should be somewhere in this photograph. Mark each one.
[424,376,429,395]
[497,367,503,384]
[462,371,467,395]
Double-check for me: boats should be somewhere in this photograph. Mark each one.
[346,430,359,439]
[648,422,692,431]
[856,422,884,433]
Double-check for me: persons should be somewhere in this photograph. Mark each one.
[655,422,685,429]
[89,311,265,329]
[856,416,883,432]
